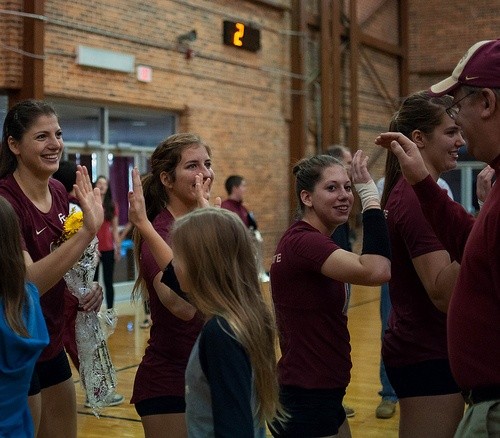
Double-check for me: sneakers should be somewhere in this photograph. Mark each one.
[83,394,124,408]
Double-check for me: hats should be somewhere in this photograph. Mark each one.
[428,37,500,97]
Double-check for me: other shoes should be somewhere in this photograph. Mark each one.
[375,399,396,418]
[140,319,152,328]
[342,404,356,417]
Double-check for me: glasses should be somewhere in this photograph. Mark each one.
[445,91,473,120]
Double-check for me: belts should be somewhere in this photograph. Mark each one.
[460,385,500,404]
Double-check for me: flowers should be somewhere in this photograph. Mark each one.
[57,210,116,402]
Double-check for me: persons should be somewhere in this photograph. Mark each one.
[127,165,292,438]
[267,150,394,437]
[128,131,223,438]
[376,89,500,438]
[51,161,126,408]
[118,172,155,329]
[90,175,121,322]
[374,37,499,437]
[0,194,50,437]
[316,144,357,419]
[218,174,262,249]
[0,98,105,438]
[371,175,455,419]
